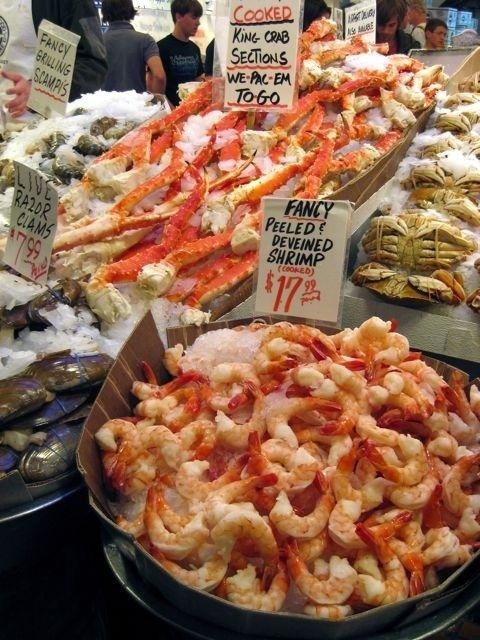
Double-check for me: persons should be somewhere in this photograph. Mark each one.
[0,2,106,128]
[98,0,166,97]
